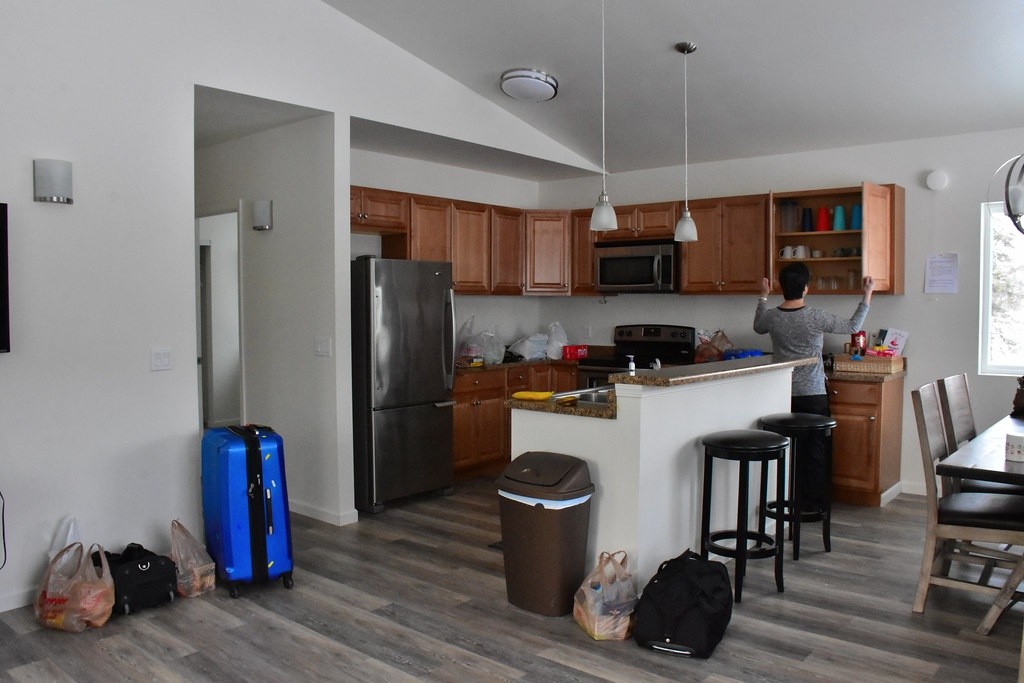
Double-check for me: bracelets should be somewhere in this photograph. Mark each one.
[758,297,767,300]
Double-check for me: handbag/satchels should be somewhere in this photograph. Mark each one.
[695,330,734,363]
[168,518,217,598]
[572,550,638,642]
[635,547,733,661]
[32,516,115,633]
[95,543,178,614]
[547,322,570,360]
[456,312,505,365]
[507,333,548,361]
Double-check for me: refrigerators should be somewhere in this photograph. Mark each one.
[351,254,456,512]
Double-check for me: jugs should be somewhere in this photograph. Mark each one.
[780,202,802,232]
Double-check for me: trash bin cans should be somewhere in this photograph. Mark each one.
[494,450,596,617]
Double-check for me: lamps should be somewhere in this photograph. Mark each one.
[588,0,619,232]
[499,67,558,100]
[252,200,273,231]
[986,153,1024,234]
[33,158,73,205]
[673,41,698,241]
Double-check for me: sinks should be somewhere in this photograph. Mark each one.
[552,392,609,405]
[597,388,615,393]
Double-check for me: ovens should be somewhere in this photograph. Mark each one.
[577,370,622,389]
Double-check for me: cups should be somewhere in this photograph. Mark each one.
[833,205,845,231]
[817,269,858,290]
[800,207,813,232]
[816,206,830,231]
[851,204,861,230]
[778,244,822,258]
[851,331,866,356]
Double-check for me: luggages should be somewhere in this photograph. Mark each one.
[202,424,296,597]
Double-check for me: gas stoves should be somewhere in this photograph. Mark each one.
[577,323,696,372]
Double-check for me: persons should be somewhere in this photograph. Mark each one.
[754,264,875,513]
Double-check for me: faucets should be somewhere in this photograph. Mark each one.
[649,358,661,370]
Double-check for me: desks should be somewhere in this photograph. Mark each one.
[935,411,1024,635]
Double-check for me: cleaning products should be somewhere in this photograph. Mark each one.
[626,354,636,377]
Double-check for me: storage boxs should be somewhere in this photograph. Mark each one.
[573,603,630,640]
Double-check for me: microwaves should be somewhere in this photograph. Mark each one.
[592,238,681,296]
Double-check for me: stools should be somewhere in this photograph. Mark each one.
[700,429,789,603]
[757,413,837,561]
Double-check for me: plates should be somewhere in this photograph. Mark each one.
[512,390,553,401]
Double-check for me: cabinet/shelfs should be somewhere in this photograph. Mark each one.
[507,364,579,397]
[766,181,906,296]
[827,377,905,508]
[382,194,492,296]
[526,210,572,296]
[491,205,526,296]
[596,200,676,241]
[350,185,410,235]
[679,193,769,295]
[573,208,618,296]
[452,368,506,478]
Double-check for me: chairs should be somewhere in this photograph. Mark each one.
[910,372,1023,613]
